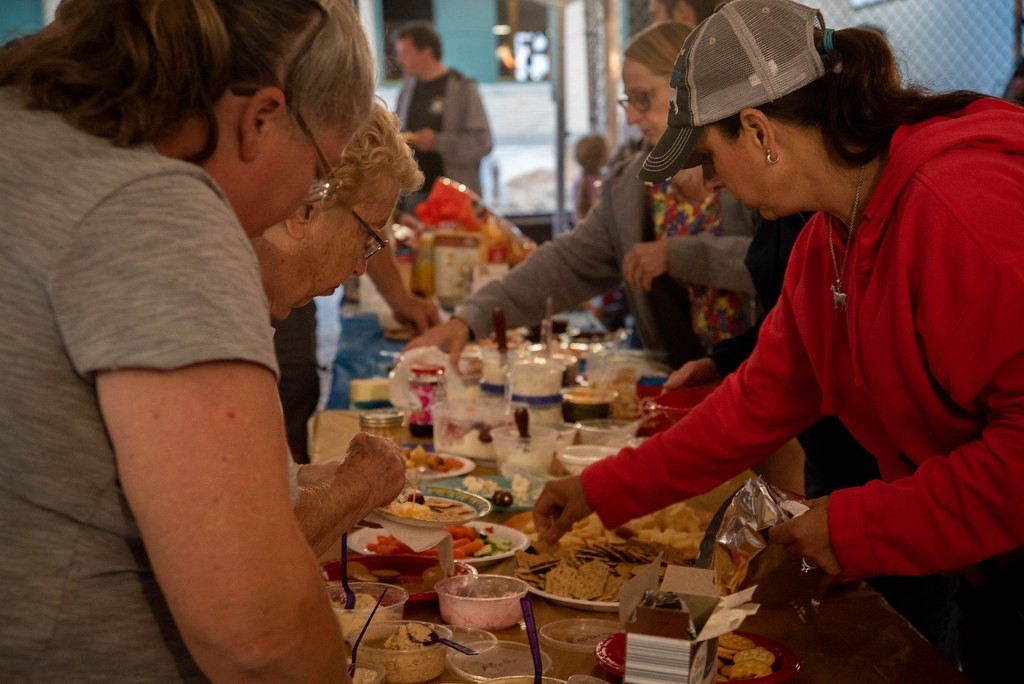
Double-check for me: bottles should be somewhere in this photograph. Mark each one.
[411,228,485,311]
[408,364,447,437]
[359,408,405,449]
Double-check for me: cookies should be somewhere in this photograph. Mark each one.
[515,537,693,603]
[709,631,776,682]
[421,497,476,515]
[346,561,451,594]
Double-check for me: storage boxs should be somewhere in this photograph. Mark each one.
[619,552,761,684]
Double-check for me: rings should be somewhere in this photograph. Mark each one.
[802,556,818,575]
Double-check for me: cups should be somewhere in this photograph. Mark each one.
[430,341,618,480]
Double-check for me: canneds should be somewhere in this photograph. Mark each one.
[358,410,405,448]
[409,365,447,437]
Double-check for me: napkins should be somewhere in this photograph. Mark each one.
[364,511,454,576]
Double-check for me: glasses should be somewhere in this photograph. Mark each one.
[335,187,389,260]
[268,87,343,205]
[617,83,665,114]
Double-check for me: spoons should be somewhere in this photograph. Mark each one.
[342,533,356,609]
[346,588,386,675]
[406,622,481,655]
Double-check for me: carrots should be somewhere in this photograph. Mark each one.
[366,523,486,559]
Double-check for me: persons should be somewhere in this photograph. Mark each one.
[0,0,376,684]
[574,135,609,218]
[385,21,492,223]
[243,104,426,556]
[269,229,440,468]
[405,0,1024,684]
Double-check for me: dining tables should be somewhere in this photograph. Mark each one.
[306,408,965,684]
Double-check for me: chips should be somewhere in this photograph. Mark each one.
[527,501,708,557]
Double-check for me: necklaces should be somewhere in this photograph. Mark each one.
[828,162,865,311]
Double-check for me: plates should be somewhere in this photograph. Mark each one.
[594,629,802,683]
[516,573,622,612]
[344,451,559,607]
[320,554,478,609]
[649,382,720,418]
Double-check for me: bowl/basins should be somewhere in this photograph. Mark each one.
[324,575,570,683]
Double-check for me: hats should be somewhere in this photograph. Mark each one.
[636,0,827,182]
